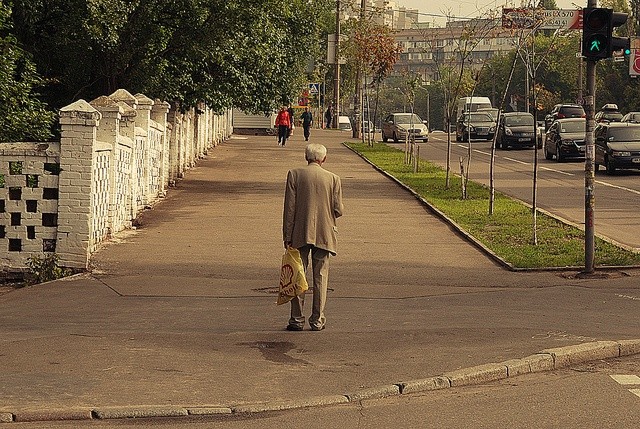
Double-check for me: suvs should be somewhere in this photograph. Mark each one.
[544,104,587,133]
[595,111,624,123]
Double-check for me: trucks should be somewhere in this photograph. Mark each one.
[456,97,492,123]
[338,115,353,131]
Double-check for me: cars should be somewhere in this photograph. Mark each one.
[381,113,429,143]
[621,113,640,123]
[543,117,594,162]
[361,121,375,132]
[592,121,640,175]
[496,111,543,150]
[455,111,497,142]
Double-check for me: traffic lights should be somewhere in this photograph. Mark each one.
[583,7,609,58]
[610,9,630,58]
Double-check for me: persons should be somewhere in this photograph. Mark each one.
[275,106,290,147]
[326,106,332,129]
[283,143,343,330]
[299,106,313,141]
